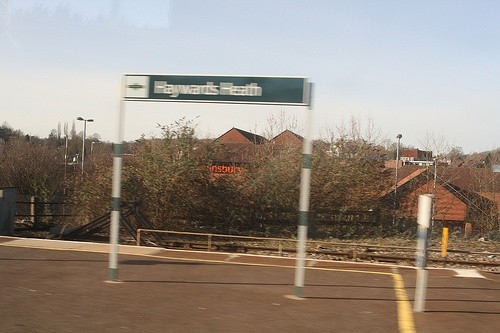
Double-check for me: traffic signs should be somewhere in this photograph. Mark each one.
[122,72,312,108]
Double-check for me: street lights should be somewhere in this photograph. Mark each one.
[392,134,402,229]
[76,116,93,181]
